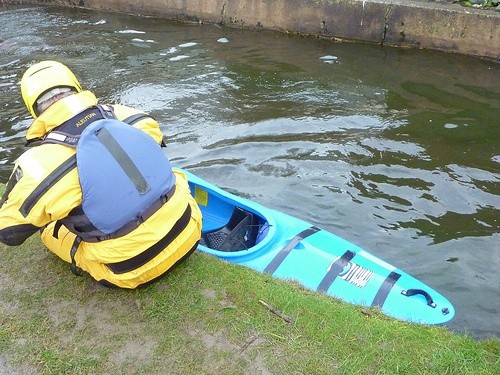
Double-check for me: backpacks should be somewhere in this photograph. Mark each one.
[41,104,176,243]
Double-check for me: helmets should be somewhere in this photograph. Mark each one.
[20,60,83,121]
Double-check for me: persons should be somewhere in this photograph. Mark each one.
[0,57,203,292]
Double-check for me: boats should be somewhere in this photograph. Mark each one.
[168,165,458,326]
